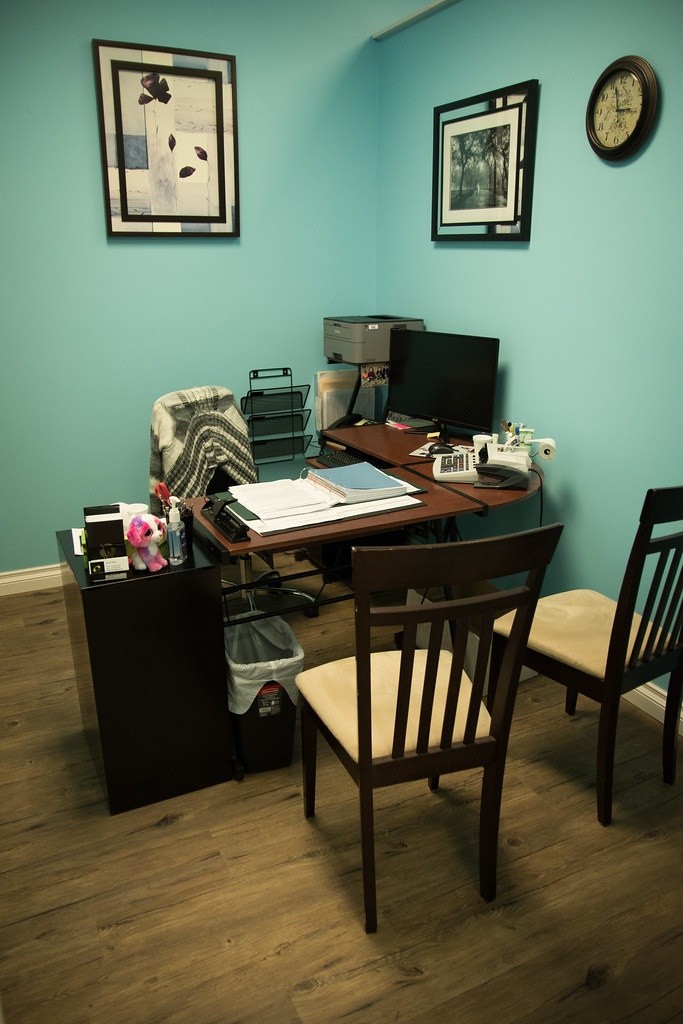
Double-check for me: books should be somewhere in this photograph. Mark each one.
[385,417,434,430]
[216,461,428,538]
[83,504,129,583]
[315,368,375,432]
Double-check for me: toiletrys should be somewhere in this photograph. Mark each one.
[165,495,188,566]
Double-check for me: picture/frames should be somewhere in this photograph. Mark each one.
[91,37,240,237]
[430,77,540,242]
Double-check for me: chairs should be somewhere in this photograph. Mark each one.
[150,386,321,617]
[294,524,567,933]
[489,483,683,828]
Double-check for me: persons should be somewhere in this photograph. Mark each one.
[365,364,389,382]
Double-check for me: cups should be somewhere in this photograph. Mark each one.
[125,503,148,529]
[473,435,492,463]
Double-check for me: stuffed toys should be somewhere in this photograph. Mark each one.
[127,514,168,572]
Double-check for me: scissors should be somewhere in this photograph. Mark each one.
[154,482,170,505]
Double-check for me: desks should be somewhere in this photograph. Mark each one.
[55,419,543,814]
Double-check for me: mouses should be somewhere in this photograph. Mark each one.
[428,442,453,454]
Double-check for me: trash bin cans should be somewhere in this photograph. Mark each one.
[225,609,305,775]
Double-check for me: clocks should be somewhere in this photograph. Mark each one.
[585,54,658,160]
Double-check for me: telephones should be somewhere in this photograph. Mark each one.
[317,413,382,447]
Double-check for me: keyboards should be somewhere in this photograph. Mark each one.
[317,449,388,470]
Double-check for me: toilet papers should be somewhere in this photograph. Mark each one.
[538,438,556,461]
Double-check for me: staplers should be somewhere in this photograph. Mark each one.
[473,463,530,491]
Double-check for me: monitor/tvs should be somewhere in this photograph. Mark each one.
[387,328,501,443]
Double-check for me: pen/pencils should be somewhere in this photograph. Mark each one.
[500,419,520,438]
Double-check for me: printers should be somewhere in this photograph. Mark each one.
[323,315,426,363]
